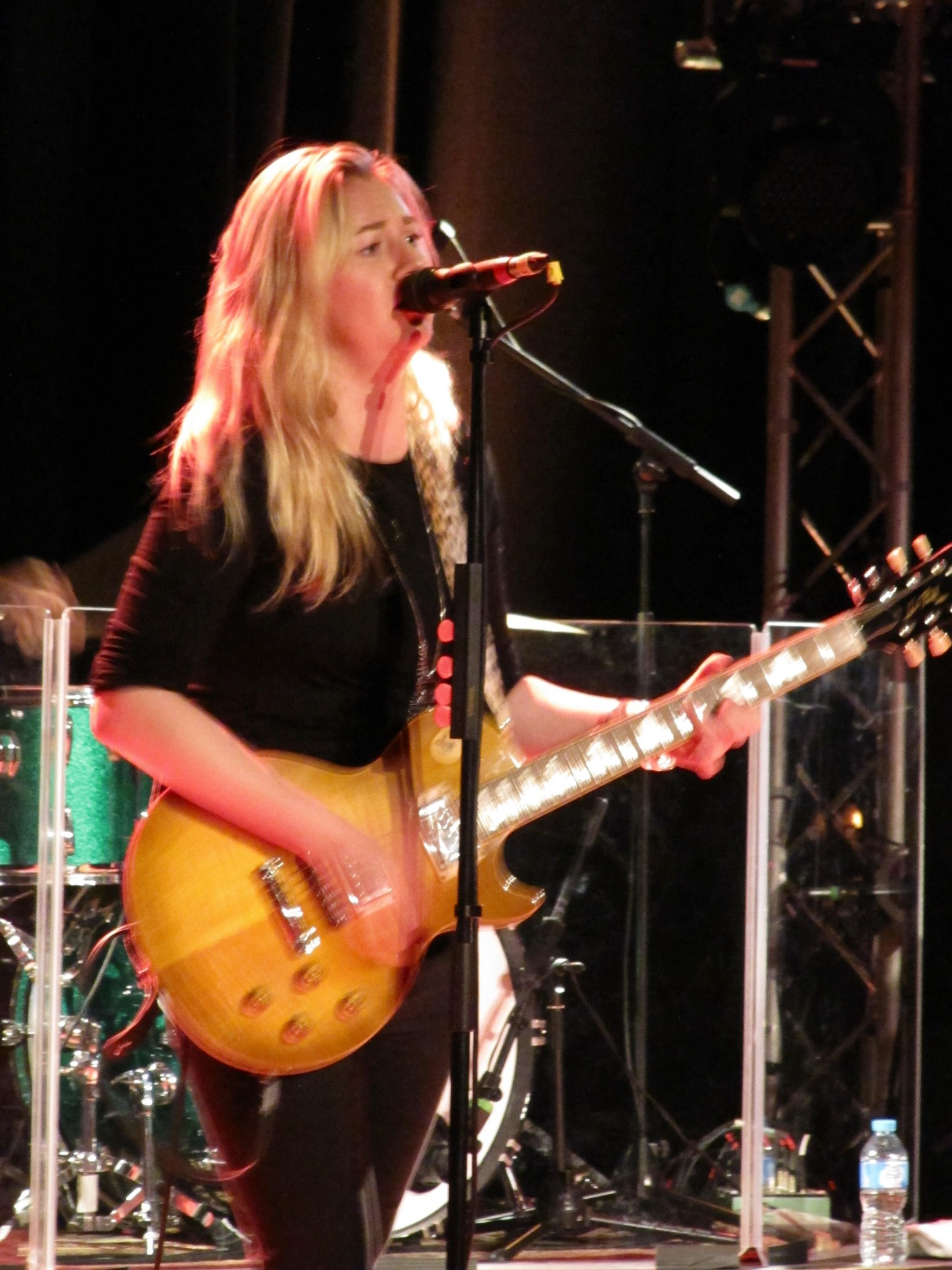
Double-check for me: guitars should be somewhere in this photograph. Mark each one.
[119,533,952,1078]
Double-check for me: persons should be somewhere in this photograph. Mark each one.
[90,144,770,1270]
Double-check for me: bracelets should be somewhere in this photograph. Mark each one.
[613,696,641,716]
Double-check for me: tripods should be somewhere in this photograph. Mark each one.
[474,339,815,1264]
[0,1014,247,1258]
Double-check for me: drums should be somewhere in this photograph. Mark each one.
[6,886,537,1237]
[0,683,153,886]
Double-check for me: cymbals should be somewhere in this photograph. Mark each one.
[505,611,587,636]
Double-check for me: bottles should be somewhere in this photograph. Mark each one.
[857,1118,914,1268]
[762,1117,774,1192]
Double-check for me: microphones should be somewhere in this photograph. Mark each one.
[398,252,550,313]
